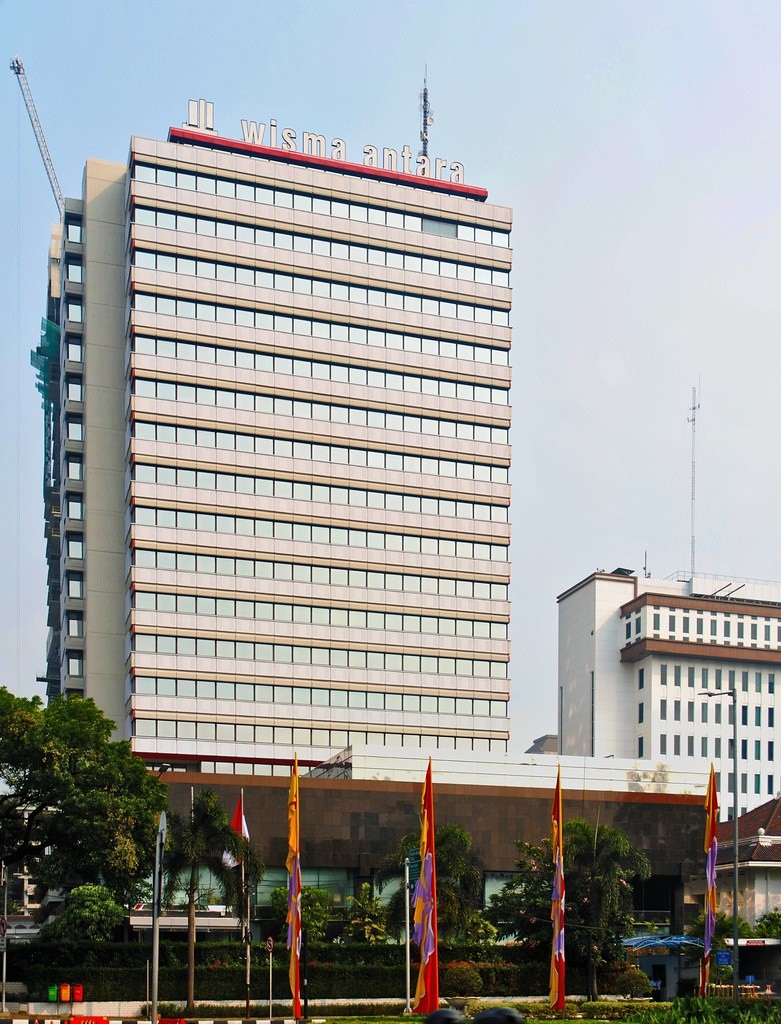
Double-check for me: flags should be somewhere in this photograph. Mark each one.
[287,752,302,1020]
[220,788,250,878]
[410,756,439,1015]
[548,766,568,1016]
[704,761,720,983]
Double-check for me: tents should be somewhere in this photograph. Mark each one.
[620,934,713,1003]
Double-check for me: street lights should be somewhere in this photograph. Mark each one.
[699,687,740,1009]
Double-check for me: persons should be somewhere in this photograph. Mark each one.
[643,974,661,1002]
[756,972,774,999]
